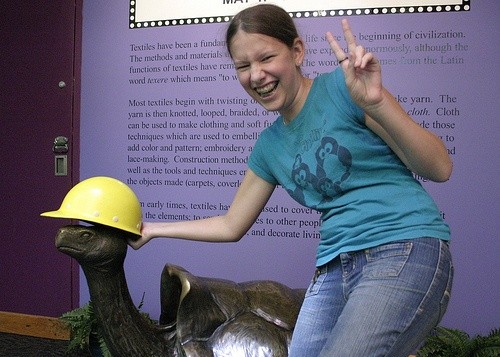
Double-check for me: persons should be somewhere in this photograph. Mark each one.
[128,4,452,357]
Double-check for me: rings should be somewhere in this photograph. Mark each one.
[339,57,348,64]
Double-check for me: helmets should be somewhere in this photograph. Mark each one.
[40,176,142,236]
